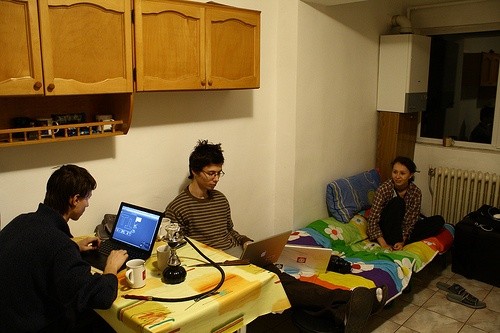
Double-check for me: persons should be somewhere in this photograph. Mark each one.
[366,156,446,252]
[163,139,294,283]
[0,165,129,333]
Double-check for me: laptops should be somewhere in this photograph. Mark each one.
[240,230,292,267]
[85,202,166,274]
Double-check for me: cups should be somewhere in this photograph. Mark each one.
[158,217,171,240]
[96,115,115,132]
[36,118,59,136]
[156,245,170,270]
[443,137,455,147]
[125,259,146,289]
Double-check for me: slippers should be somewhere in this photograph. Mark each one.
[436,281,465,296]
[368,284,389,316]
[342,287,373,333]
[447,291,486,309]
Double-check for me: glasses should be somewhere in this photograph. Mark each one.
[199,169,225,178]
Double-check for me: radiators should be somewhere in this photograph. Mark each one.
[431,167,500,226]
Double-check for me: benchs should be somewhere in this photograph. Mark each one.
[276,213,455,305]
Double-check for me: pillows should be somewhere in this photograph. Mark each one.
[326,169,382,224]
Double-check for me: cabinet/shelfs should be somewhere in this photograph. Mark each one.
[132,0,261,92]
[377,111,418,183]
[0,0,133,95]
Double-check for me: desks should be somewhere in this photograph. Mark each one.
[70,233,291,333]
